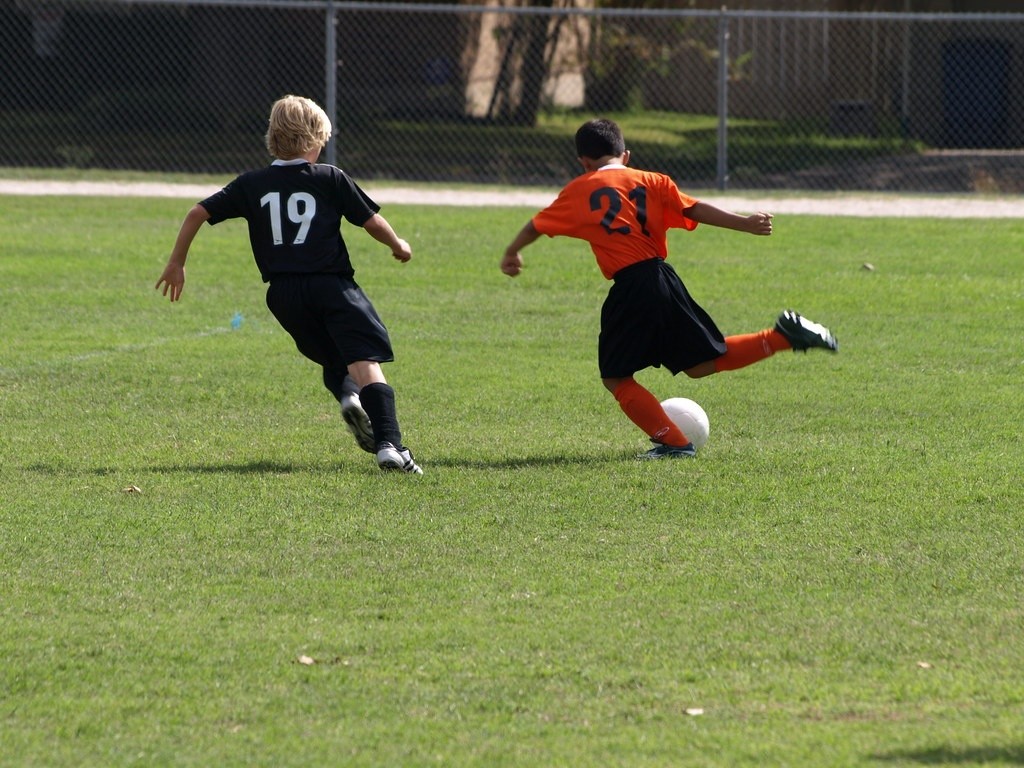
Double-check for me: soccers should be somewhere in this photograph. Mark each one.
[660,398,709,450]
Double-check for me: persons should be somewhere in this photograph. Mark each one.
[154,95,424,479]
[499,118,841,459]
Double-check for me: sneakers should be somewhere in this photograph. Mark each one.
[341,396,377,454]
[775,310,837,355]
[637,438,697,458]
[375,441,424,476]
